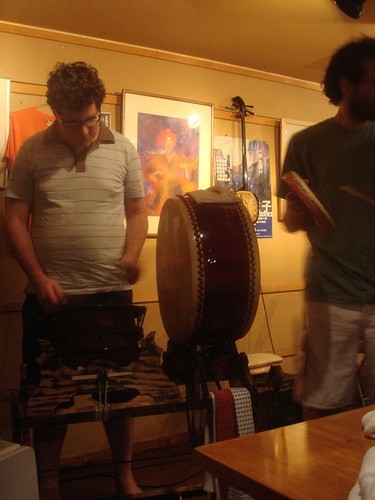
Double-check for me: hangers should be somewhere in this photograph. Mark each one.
[34,103,54,118]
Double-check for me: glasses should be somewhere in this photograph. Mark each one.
[50,104,101,128]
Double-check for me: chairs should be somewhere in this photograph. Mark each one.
[355,353,368,407]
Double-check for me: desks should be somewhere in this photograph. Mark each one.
[194,405,374,500]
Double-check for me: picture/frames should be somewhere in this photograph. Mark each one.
[279,117,316,223]
[121,88,215,238]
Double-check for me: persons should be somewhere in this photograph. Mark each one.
[274,38,375,419]
[7,62,144,500]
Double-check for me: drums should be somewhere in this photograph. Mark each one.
[281,170,336,242]
[155,186,262,346]
